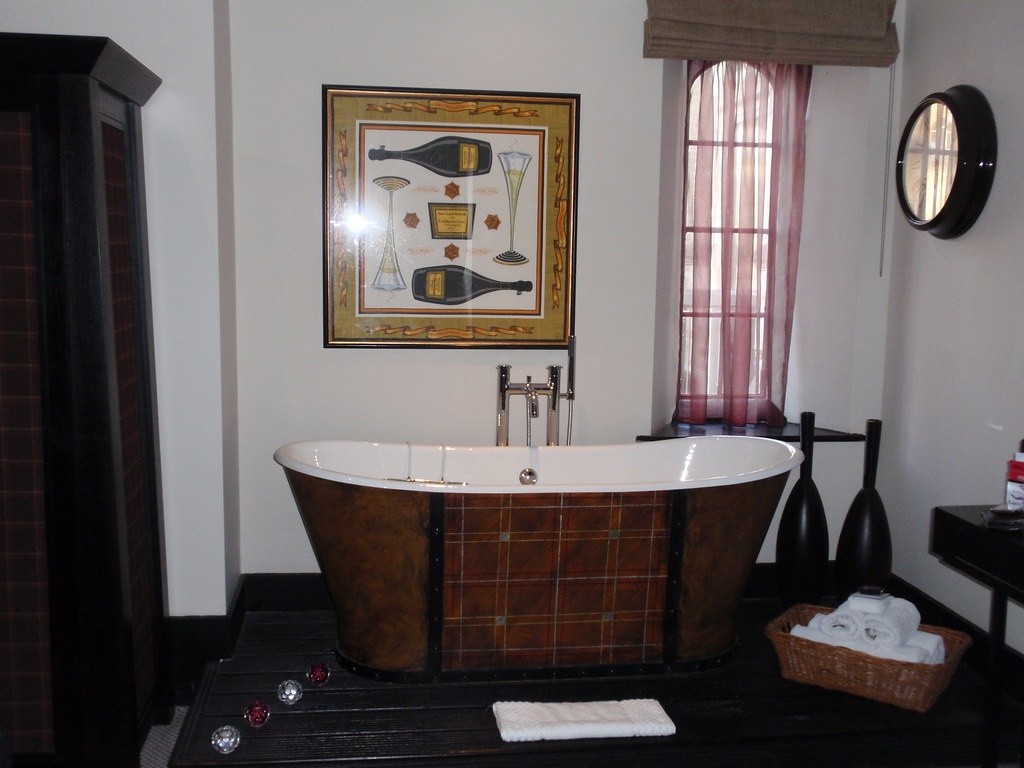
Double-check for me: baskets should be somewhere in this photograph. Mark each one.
[763,604,972,713]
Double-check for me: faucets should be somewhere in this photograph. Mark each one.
[522,376,543,419]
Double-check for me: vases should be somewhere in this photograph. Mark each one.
[776,412,829,579]
[837,420,893,590]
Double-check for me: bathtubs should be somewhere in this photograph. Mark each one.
[270,430,807,680]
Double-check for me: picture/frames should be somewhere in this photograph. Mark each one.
[320,82,580,351]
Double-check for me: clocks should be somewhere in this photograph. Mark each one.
[895,84,999,241]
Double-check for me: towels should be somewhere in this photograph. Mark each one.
[491,698,678,743]
[788,591,947,667]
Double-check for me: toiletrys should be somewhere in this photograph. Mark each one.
[1005,452,1024,513]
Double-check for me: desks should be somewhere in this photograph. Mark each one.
[931,504,1024,681]
